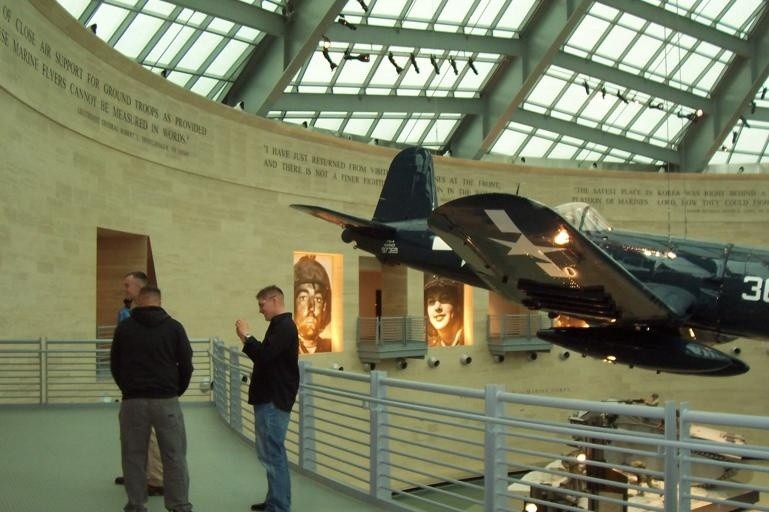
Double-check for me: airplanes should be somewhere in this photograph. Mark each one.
[291,144,769,375]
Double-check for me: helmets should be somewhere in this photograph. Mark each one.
[293,256,331,323]
[424,271,461,295]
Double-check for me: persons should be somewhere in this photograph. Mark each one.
[561,452,587,494]
[114,271,165,496]
[631,460,664,498]
[292,255,331,355]
[236,284,300,512]
[644,393,661,425]
[424,272,465,347]
[109,284,194,512]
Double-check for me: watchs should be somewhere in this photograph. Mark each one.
[244,334,251,342]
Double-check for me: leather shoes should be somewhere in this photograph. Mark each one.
[115,476,124,484]
[147,485,164,496]
[251,503,266,511]
